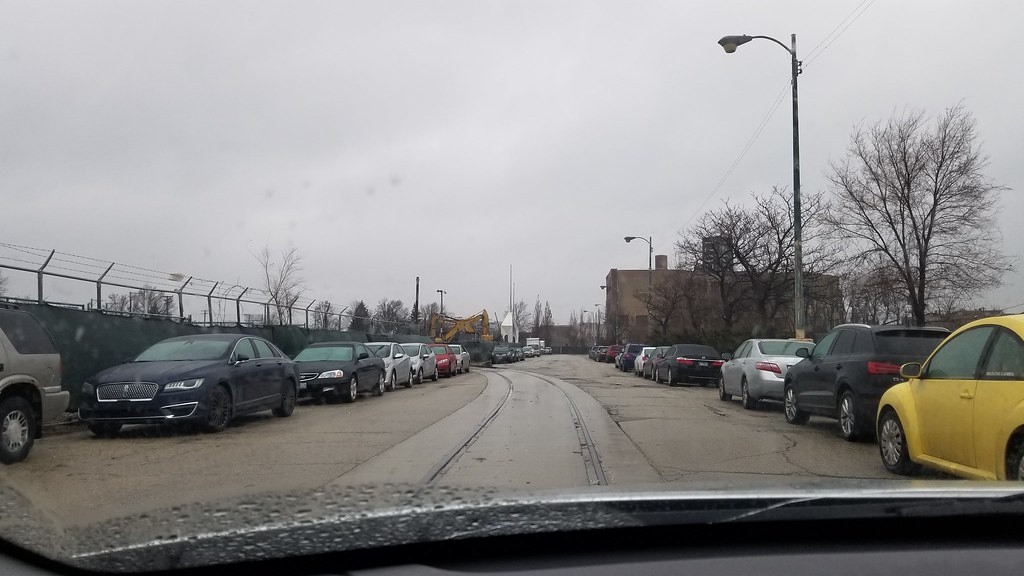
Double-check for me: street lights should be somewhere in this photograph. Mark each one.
[716,31,805,340]
[582,283,621,346]
[436,289,447,314]
[624,235,653,345]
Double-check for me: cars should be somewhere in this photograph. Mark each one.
[875,313,1024,484]
[587,343,649,372]
[718,339,816,410]
[642,345,672,381]
[491,336,555,365]
[428,342,458,377]
[634,346,655,376]
[447,344,471,373]
[399,342,438,382]
[76,333,302,439]
[655,343,727,388]
[362,341,415,390]
[292,340,387,404]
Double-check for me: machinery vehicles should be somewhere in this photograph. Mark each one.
[428,308,493,346]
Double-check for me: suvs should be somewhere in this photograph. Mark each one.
[784,322,953,442]
[0,299,71,464]
